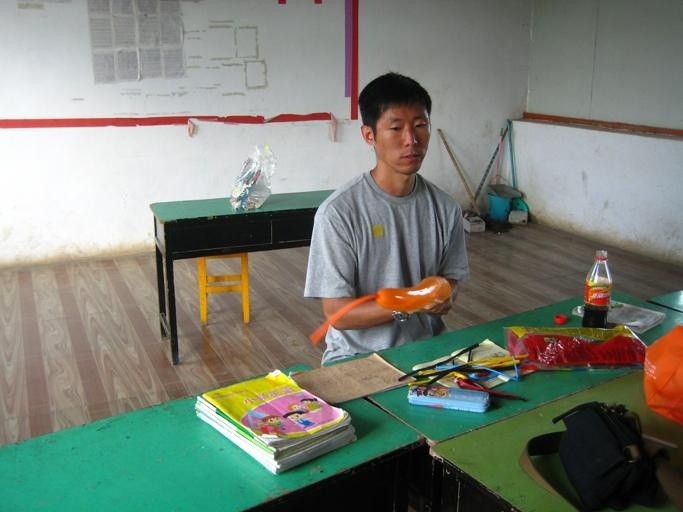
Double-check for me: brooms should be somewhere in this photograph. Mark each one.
[505,118,529,225]
[437,128,509,233]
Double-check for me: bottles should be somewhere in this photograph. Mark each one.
[580,249,611,327]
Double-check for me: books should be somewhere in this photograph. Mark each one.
[194,368,358,475]
[412,338,535,390]
[572,298,666,334]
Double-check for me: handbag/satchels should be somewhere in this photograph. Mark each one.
[552,400,658,511]
[642,325,683,425]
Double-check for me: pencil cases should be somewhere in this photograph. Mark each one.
[407,385,491,413]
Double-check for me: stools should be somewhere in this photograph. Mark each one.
[195,251,250,327]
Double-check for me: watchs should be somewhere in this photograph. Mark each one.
[391,309,409,323]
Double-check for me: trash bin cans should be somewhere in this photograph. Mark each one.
[487,193,512,221]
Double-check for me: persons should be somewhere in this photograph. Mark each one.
[303,70,471,364]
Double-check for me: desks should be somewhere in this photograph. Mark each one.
[147,189,344,367]
[0,360,426,511]
[325,285,683,511]
